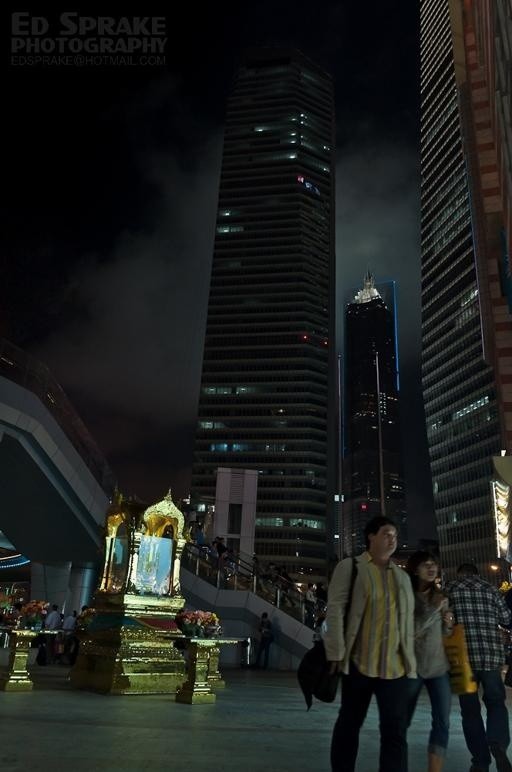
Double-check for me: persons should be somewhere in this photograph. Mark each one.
[253,557,296,608]
[195,524,229,581]
[32,605,89,666]
[446,563,510,772]
[406,551,453,770]
[325,519,418,771]
[305,581,328,631]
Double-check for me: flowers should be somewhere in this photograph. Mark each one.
[175,609,220,638]
[0,593,51,632]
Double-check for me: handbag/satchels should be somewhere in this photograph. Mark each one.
[296,639,341,712]
[443,626,477,695]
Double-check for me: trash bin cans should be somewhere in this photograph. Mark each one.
[242,637,257,666]
